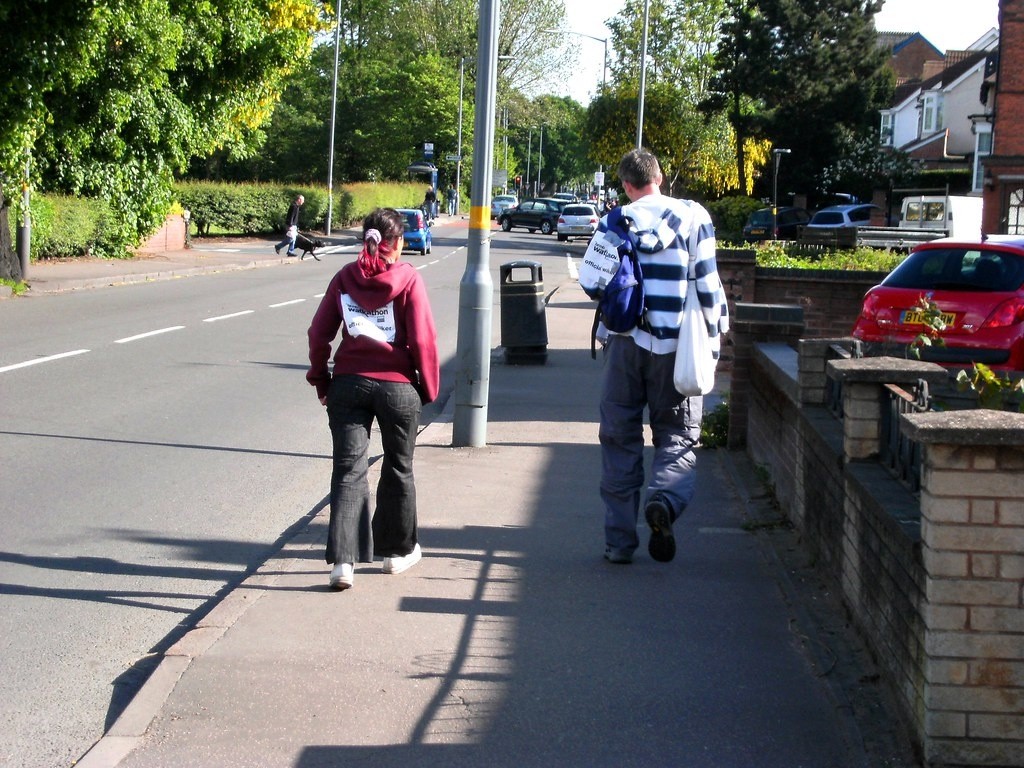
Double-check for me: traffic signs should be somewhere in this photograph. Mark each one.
[446,155,461,160]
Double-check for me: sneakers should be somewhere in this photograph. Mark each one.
[328,562,354,589]
[383,543,422,573]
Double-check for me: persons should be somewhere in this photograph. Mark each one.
[600,197,619,218]
[425,186,436,220]
[306,207,440,589]
[447,184,457,217]
[579,149,729,565]
[275,194,305,257]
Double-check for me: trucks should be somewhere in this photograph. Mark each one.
[857,195,982,250]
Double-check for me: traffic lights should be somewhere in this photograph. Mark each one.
[515,176,522,189]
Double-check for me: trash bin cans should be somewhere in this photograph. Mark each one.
[501,261,550,367]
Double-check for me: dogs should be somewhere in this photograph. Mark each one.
[294,234,325,261]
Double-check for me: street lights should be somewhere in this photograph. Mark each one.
[526,124,538,197]
[455,56,517,216]
[544,29,608,97]
[773,148,791,239]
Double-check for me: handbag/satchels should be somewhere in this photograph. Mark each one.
[674,201,715,396]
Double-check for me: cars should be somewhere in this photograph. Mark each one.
[556,204,601,241]
[742,205,809,238]
[396,209,435,256]
[581,194,588,200]
[805,203,889,239]
[491,194,518,219]
[851,233,1024,412]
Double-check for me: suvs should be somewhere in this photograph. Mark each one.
[553,193,578,203]
[496,197,570,235]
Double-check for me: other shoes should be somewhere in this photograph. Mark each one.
[275,245,279,254]
[645,499,676,563]
[603,547,632,563]
[287,252,297,257]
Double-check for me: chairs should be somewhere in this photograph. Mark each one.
[975,257,1002,276]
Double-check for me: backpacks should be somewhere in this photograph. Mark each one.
[590,207,648,335]
